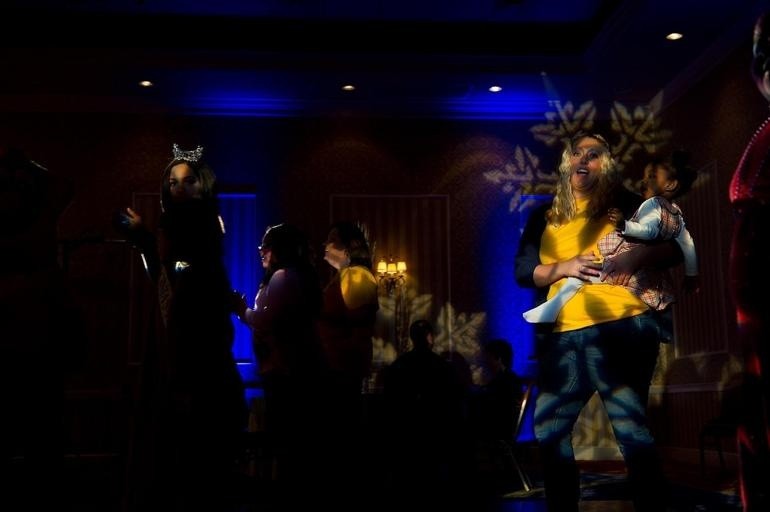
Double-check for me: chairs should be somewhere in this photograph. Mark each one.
[505,381,534,492]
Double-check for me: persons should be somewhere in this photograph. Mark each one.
[112,13,769,512]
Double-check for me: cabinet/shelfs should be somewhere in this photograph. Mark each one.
[657,427,759,512]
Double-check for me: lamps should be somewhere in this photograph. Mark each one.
[376,245,407,297]
